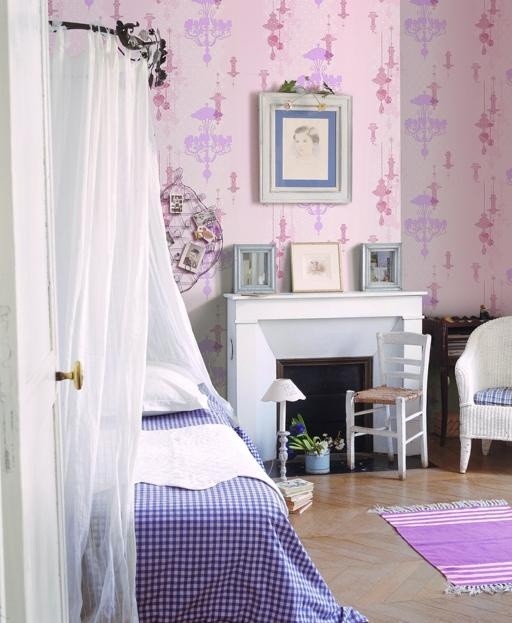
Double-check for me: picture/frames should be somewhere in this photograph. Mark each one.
[256,90,354,204]
[288,240,345,293]
[177,239,207,275]
[230,242,277,295]
[360,242,404,292]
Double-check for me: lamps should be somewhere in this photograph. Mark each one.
[260,375,308,484]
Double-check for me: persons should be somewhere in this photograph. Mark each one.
[283,123,320,179]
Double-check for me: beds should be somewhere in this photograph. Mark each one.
[52,351,371,623]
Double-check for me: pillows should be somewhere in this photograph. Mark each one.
[142,360,210,418]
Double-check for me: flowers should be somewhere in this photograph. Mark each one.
[285,414,348,456]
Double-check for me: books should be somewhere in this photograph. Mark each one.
[275,477,314,515]
[448,333,470,356]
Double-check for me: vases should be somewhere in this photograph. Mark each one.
[303,447,333,475]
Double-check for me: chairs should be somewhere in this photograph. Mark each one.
[344,327,433,480]
[452,310,512,476]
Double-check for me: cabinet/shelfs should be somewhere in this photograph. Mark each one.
[423,314,506,448]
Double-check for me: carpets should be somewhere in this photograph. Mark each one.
[367,495,512,599]
